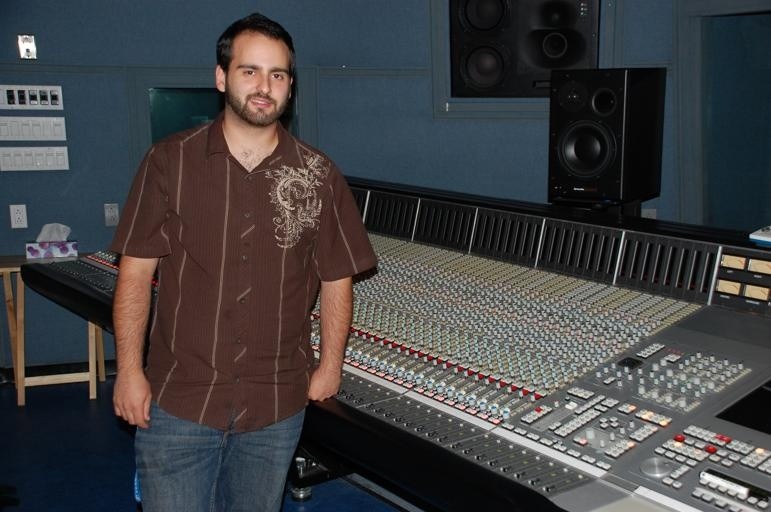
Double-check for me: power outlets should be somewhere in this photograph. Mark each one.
[105,203,121,228]
[10,204,27,230]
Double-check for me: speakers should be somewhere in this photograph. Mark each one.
[546,63,668,204]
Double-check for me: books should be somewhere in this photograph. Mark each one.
[748,226,771,244]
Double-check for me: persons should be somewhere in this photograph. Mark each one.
[109,12,382,512]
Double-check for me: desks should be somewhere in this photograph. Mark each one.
[0,252,107,408]
